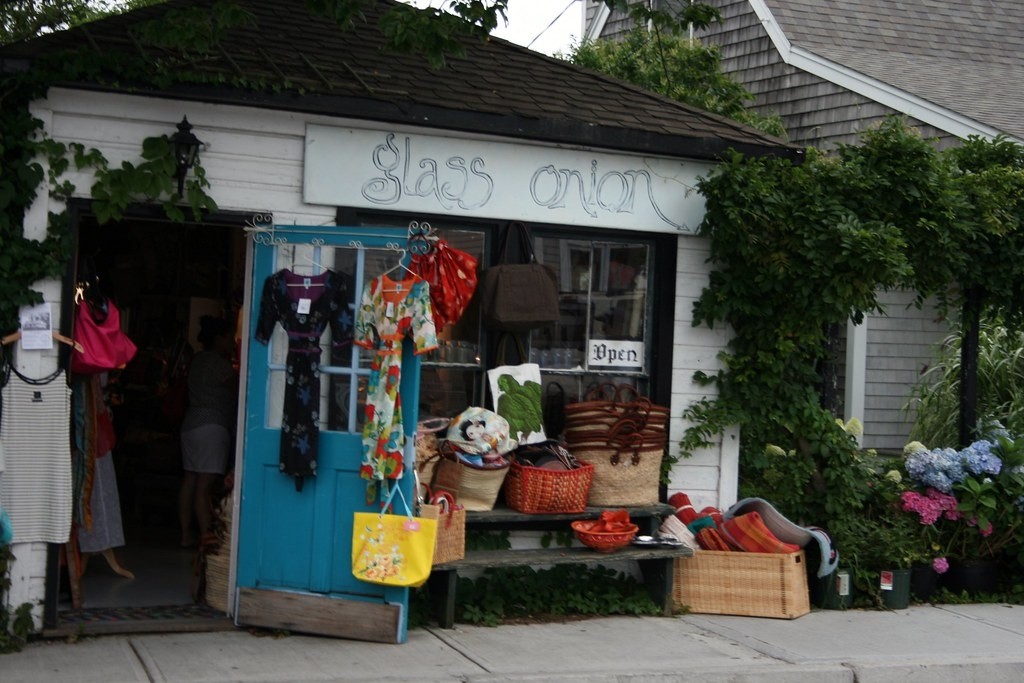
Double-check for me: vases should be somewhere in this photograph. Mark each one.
[815,554,1015,610]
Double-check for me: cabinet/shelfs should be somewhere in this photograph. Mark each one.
[427,502,693,630]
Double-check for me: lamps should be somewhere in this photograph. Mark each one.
[167,116,205,200]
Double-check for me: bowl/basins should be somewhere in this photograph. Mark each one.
[571,521,639,553]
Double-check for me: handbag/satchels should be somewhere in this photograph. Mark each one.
[401,231,478,337]
[482,221,560,329]
[71,280,139,375]
[487,333,549,446]
[350,480,438,588]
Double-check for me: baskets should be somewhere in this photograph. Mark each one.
[563,379,669,508]
[429,438,510,512]
[506,461,594,514]
[572,520,638,551]
[412,418,450,502]
[667,552,811,620]
[413,481,466,567]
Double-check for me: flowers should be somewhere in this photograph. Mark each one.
[763,417,1024,574]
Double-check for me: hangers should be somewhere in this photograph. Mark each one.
[280,243,328,287]
[1,332,84,354]
[382,249,423,291]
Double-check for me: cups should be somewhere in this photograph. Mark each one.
[530,348,575,369]
